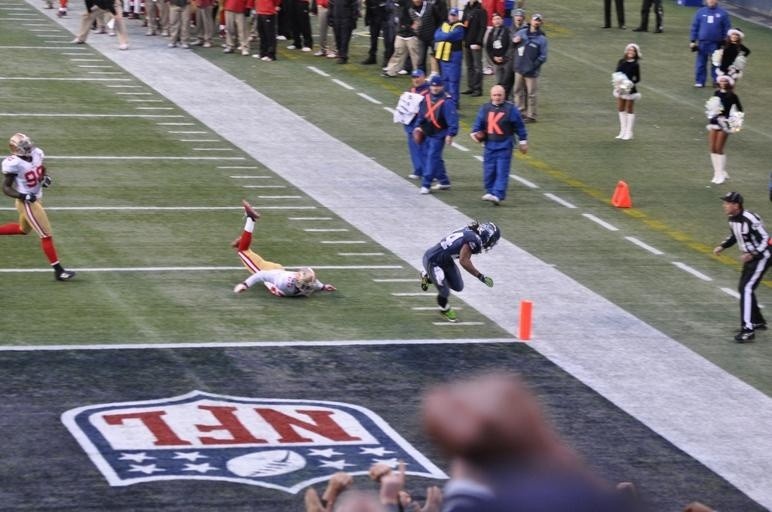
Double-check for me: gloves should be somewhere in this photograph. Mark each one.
[40,175,52,188]
[478,273,494,288]
[18,192,37,203]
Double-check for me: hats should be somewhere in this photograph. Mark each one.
[716,74,735,89]
[727,28,744,39]
[449,7,459,17]
[719,191,744,203]
[411,69,425,78]
[424,75,442,86]
[624,43,642,59]
[532,13,543,21]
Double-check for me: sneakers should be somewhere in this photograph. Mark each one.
[522,118,535,123]
[735,328,755,342]
[490,195,500,206]
[241,198,260,220]
[431,184,450,190]
[419,186,432,195]
[407,174,419,181]
[54,270,76,281]
[439,308,458,322]
[741,321,768,330]
[419,270,429,292]
[694,84,704,87]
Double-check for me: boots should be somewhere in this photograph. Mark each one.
[709,153,730,184]
[621,113,635,141]
[616,111,626,139]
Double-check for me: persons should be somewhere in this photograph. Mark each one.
[422,373,654,512]
[405,71,430,180]
[714,192,772,343]
[233,200,337,298]
[471,85,528,206]
[712,28,750,88]
[613,43,641,141]
[690,0,730,87]
[413,75,459,194]
[600,0,626,29]
[421,222,500,322]
[632,0,666,32]
[304,460,443,512]
[683,502,716,512]
[43,0,547,122]
[0,133,76,280]
[706,76,744,184]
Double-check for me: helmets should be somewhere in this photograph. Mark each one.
[477,221,500,252]
[296,265,316,292]
[8,132,33,158]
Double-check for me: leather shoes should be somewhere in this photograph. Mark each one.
[460,89,472,94]
[471,91,482,97]
